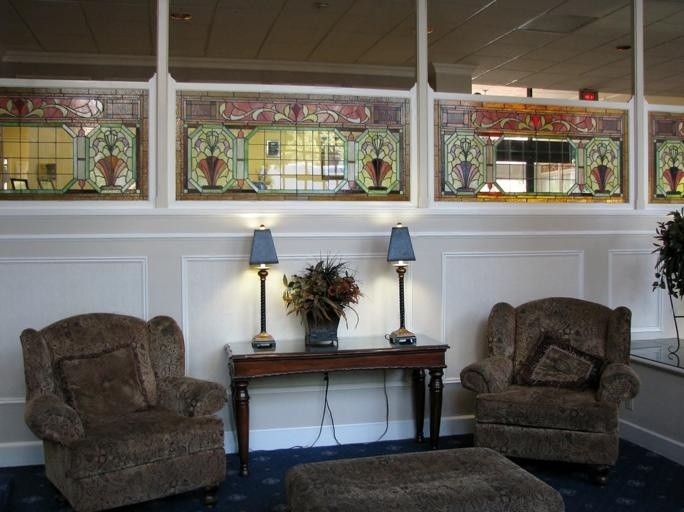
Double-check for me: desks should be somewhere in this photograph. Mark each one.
[224,332,450,477]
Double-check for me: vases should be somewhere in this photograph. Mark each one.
[299,311,339,353]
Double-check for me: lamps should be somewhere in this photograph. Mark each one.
[249,225,279,349]
[387,222,417,345]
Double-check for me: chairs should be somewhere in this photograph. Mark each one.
[20,313,226,511]
[460,297,641,485]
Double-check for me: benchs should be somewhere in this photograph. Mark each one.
[285,446,565,511]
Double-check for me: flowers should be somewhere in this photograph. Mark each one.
[282,249,364,331]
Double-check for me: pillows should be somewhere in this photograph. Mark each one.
[54,344,148,422]
[522,337,602,388]
